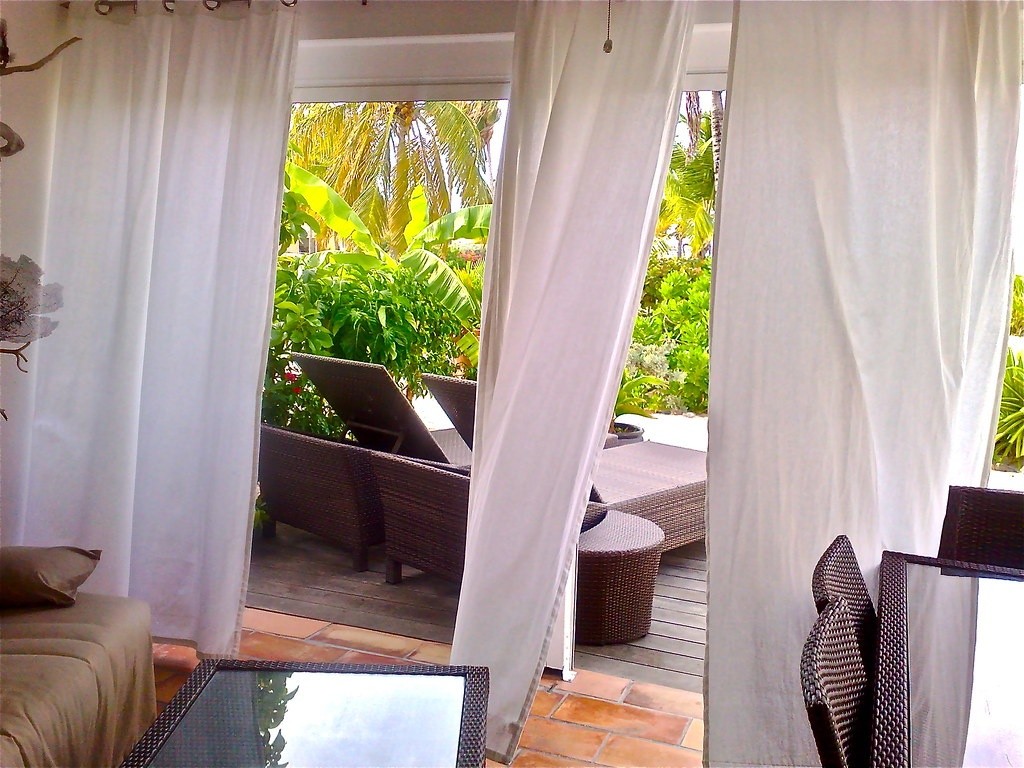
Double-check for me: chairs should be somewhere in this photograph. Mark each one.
[800,595,869,768]
[371,372,705,595]
[811,535,877,674]
[259,352,645,572]
[937,485,1024,569]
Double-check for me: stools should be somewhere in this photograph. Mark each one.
[577,509,666,645]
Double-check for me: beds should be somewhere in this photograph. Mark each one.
[0,592,157,768]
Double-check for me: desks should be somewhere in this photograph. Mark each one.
[115,658,490,768]
[869,550,1024,767]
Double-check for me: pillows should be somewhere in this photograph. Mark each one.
[0,545,103,607]
